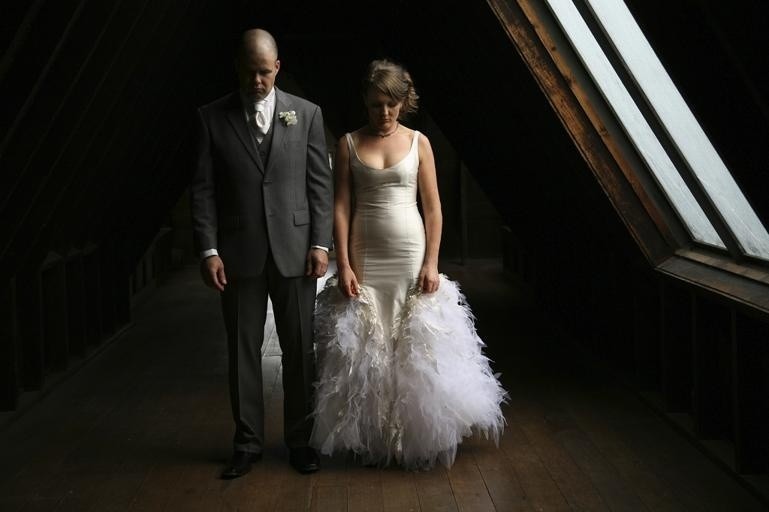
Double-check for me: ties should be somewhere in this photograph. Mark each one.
[249,100,270,145]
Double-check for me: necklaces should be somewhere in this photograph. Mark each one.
[369,121,399,137]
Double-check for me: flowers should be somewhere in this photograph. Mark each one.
[279,110,298,127]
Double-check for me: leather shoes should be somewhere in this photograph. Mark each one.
[290,447,320,470]
[221,458,256,477]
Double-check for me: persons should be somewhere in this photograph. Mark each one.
[304,60,510,473]
[188,29,335,480]
